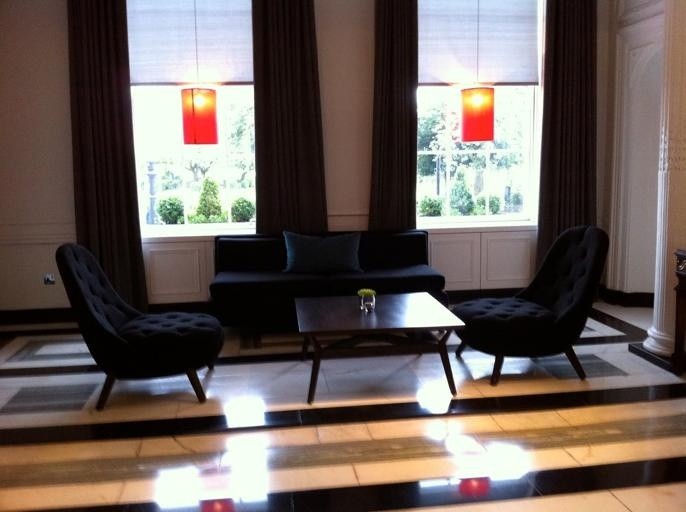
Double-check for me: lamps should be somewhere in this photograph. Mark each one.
[459,477,490,498]
[182,0,217,144]
[462,1,495,141]
[198,464,235,511]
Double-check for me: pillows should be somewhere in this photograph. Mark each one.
[281,230,363,274]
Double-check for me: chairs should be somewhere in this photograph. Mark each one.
[450,225,609,386]
[55,243,225,411]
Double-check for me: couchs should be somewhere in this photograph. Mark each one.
[210,230,450,333]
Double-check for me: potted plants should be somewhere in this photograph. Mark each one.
[357,289,376,309]
